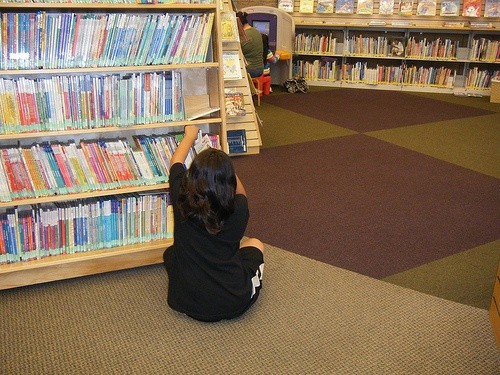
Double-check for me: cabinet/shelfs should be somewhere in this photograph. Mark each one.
[0,0,229,292]
[277,0,500,96]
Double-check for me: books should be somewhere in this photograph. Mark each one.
[0,0,263,266]
[278,0,500,90]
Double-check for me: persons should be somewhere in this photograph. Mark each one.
[261,33,281,93]
[162,125,265,322]
[236,11,264,78]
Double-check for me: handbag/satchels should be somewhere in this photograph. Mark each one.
[282,77,309,94]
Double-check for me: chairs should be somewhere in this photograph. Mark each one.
[252,73,272,95]
